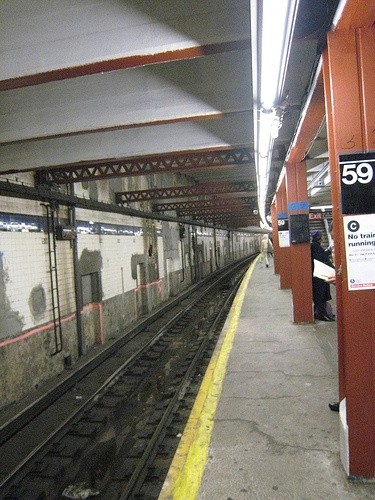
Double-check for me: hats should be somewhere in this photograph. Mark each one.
[311,231,321,240]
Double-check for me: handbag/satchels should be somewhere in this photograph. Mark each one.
[267,244,272,253]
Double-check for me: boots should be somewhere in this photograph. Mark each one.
[313,302,335,321]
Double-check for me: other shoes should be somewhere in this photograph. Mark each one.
[266,265,268,268]
[329,402,340,411]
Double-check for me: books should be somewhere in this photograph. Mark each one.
[312,258,335,281]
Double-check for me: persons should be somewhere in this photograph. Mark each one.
[324,275,339,411]
[260,234,274,268]
[310,229,335,321]
